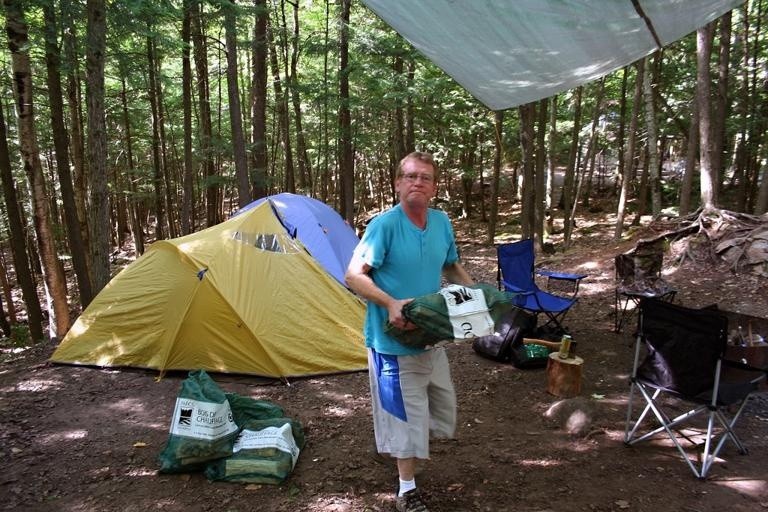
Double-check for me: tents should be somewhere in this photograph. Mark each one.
[47,199,370,388]
[232,191,360,291]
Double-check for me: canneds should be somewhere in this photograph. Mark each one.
[558,334,573,359]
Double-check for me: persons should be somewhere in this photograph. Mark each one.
[344,151,476,510]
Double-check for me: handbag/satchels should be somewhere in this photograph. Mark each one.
[473,306,538,365]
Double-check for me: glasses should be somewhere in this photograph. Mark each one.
[404,174,433,183]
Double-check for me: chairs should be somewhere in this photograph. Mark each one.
[622,298,768,480]
[495,238,589,335]
[614,253,677,333]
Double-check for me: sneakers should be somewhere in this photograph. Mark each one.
[395,488,430,512]
